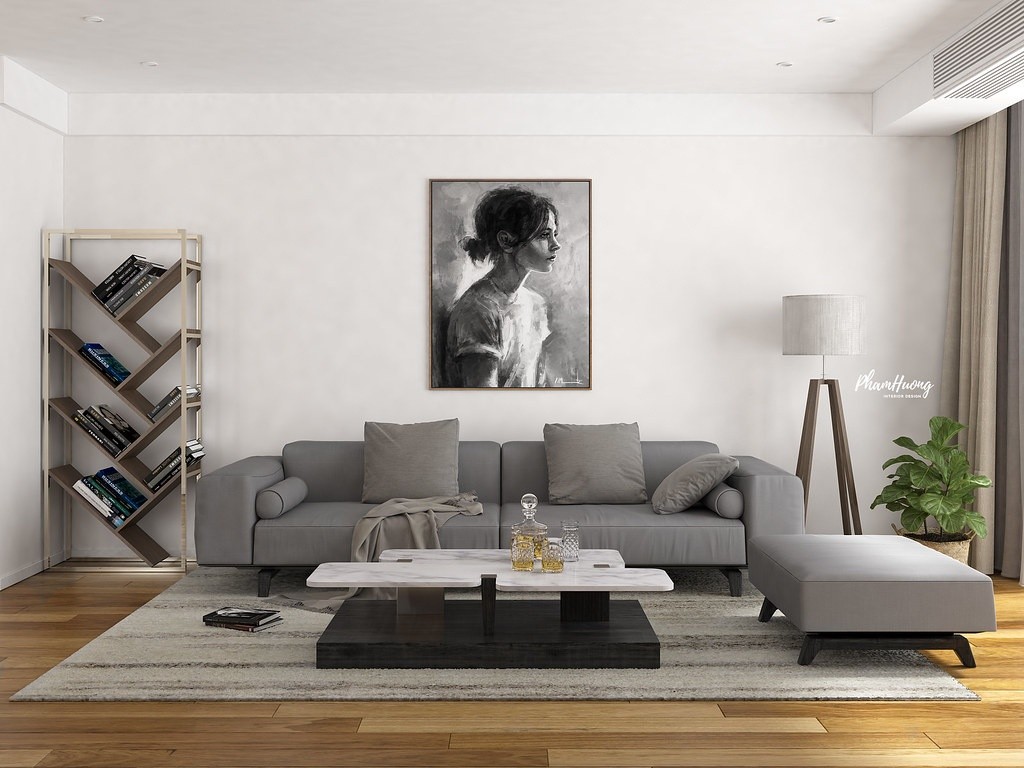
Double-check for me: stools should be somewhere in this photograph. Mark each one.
[748,535,997,669]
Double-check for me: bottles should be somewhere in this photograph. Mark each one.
[510,493,548,561]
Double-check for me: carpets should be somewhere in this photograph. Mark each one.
[8,562,983,703]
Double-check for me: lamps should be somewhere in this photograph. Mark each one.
[781,294,863,536]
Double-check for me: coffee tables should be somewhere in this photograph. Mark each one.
[306,548,675,670]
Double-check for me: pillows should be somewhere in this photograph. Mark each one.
[361,418,460,504]
[543,422,647,505]
[652,453,739,516]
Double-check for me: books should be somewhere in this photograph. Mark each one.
[91,253,167,317]
[72,467,148,529]
[74,403,141,458]
[202,606,283,633]
[144,439,206,497]
[147,384,198,425]
[78,341,132,390]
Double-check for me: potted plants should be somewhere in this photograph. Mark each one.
[869,415,994,565]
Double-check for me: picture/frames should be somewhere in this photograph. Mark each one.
[427,178,593,391]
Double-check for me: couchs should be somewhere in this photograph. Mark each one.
[193,441,805,597]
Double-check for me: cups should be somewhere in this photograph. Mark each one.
[511,537,534,572]
[559,520,581,562]
[540,537,565,573]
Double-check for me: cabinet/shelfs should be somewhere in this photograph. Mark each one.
[43,227,203,572]
